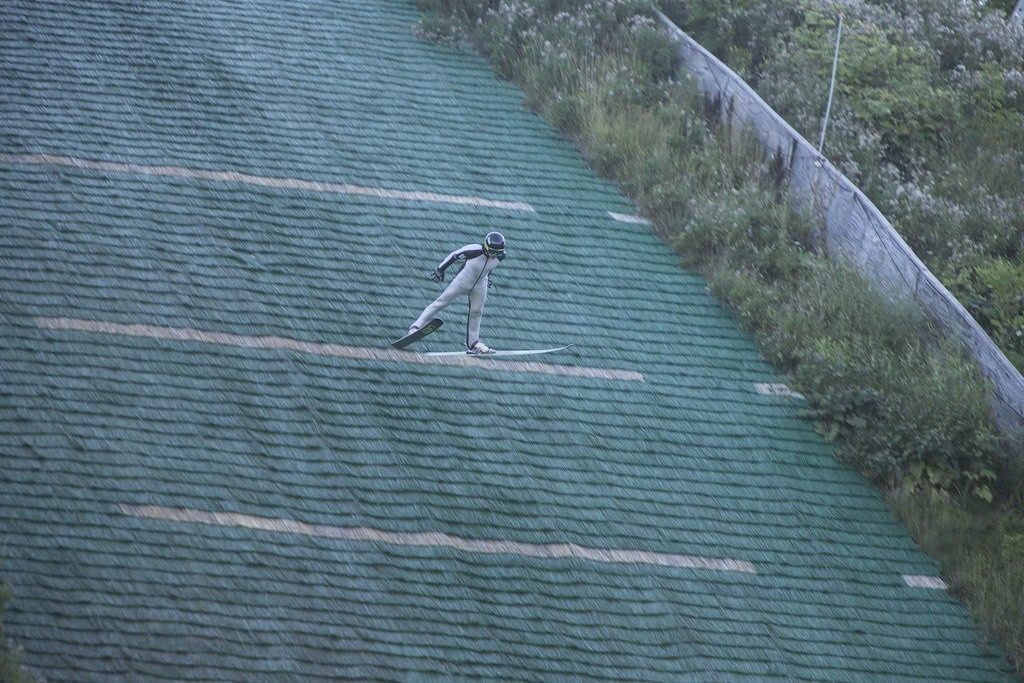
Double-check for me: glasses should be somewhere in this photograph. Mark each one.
[485,249,505,256]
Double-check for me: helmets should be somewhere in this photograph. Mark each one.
[484,232,505,251]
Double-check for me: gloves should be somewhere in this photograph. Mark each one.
[487,276,492,289]
[430,268,446,283]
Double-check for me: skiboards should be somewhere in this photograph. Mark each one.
[390,317,574,355]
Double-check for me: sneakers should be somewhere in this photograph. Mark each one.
[470,342,495,353]
[408,328,419,335]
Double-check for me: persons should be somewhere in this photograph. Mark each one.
[407,232,506,352]
